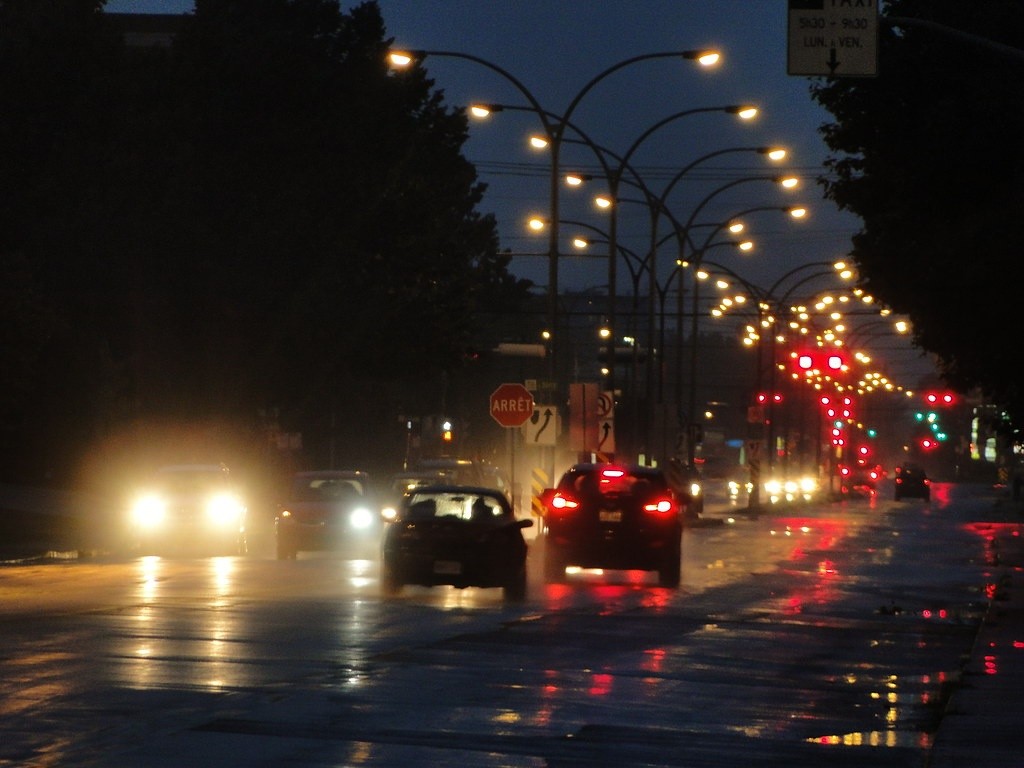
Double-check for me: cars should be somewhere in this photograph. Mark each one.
[134,464,245,555]
[540,463,683,591]
[638,448,931,510]
[378,486,535,605]
[274,469,382,562]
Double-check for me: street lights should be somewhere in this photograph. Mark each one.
[375,40,912,517]
